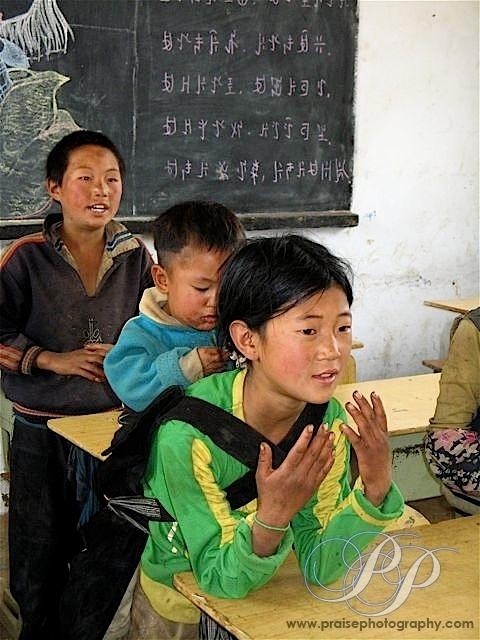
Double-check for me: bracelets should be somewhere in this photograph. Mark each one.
[250,515,292,535]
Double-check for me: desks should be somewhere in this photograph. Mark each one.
[13,298,480,640]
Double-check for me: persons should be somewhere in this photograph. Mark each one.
[424,304,480,519]
[104,231,406,640]
[99,202,248,415]
[0,131,157,638]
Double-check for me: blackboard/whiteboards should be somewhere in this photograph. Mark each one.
[0,1,360,239]
